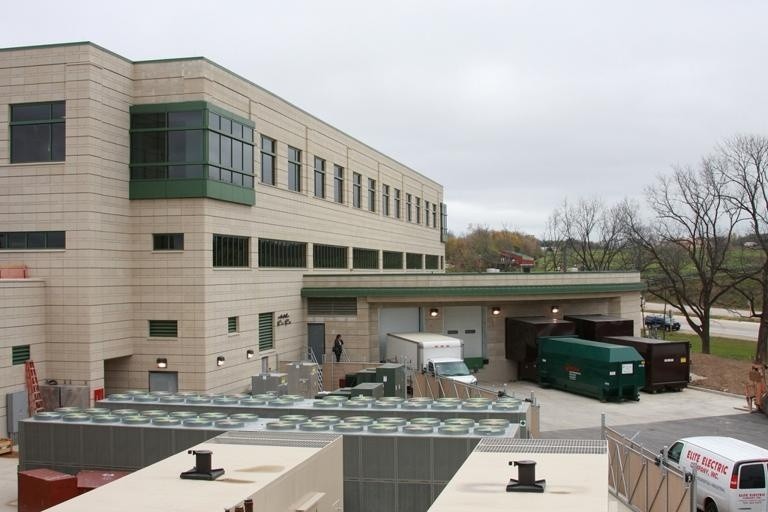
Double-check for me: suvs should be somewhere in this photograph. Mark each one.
[644,315,680,331]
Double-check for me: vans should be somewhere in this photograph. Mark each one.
[654,435,768,512]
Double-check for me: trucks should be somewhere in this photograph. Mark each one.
[385,331,478,396]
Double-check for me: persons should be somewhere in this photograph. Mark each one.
[333,334,344,362]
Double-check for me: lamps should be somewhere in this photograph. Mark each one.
[157,358,167,368]
[492,307,500,315]
[217,357,225,366]
[552,306,560,313]
[247,350,254,359]
[430,309,438,316]
